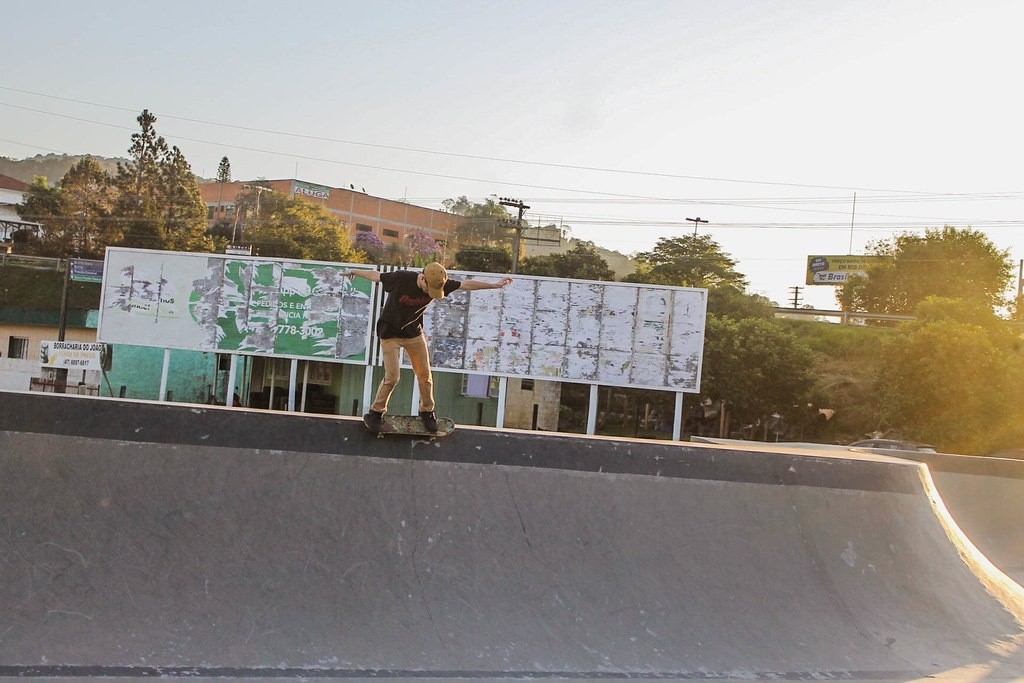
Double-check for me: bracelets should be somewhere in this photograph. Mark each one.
[350,269,352,274]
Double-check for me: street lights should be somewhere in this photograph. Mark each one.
[684,215,709,247]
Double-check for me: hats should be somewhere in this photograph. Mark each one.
[424,262,448,300]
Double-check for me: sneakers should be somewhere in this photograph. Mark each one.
[369,409,385,433]
[419,410,438,431]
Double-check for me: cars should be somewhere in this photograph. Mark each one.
[848,438,937,454]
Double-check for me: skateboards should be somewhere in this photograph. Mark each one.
[363,413,457,441]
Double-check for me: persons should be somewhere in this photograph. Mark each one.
[349,261,514,434]
